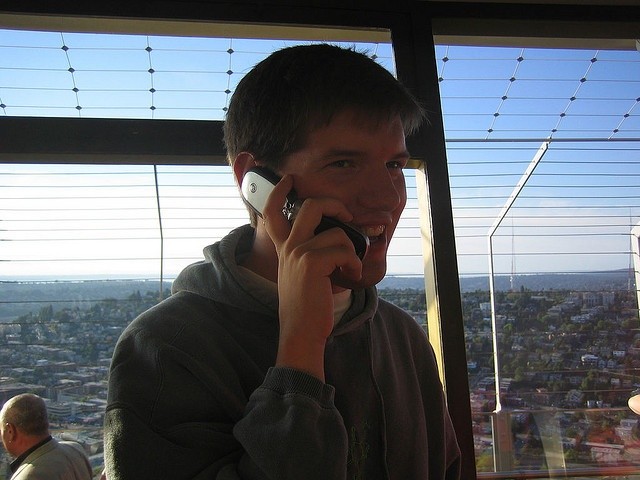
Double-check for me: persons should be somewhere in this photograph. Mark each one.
[102,44,462,480]
[0,393,93,480]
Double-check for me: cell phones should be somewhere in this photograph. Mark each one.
[237,166,370,271]
